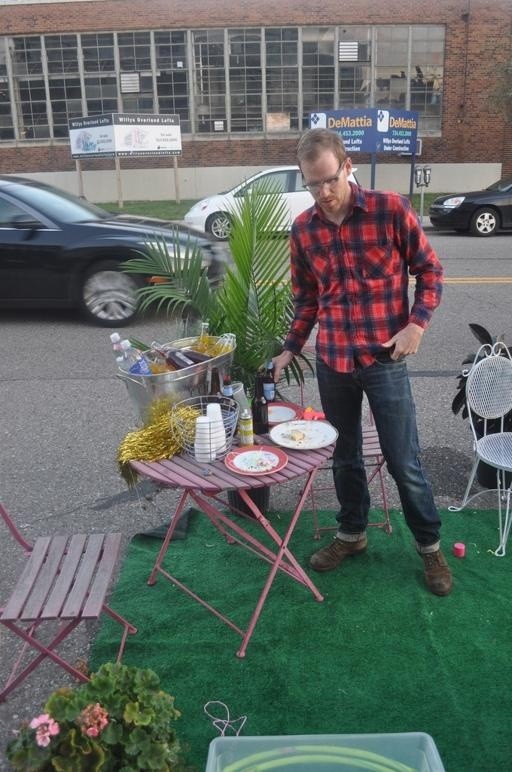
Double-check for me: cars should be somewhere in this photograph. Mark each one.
[184,165,362,242]
[428,178,511,237]
[0,176,222,326]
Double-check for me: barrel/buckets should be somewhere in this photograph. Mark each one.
[116,336,237,426]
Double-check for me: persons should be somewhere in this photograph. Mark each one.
[258,128,453,597]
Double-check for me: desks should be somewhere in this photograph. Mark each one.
[129,398,334,657]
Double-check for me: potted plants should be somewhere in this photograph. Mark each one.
[451,323,511,489]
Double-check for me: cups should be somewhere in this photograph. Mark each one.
[231,382,249,411]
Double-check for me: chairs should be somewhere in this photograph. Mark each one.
[0,498,138,701]
[448,342,511,557]
[300,344,393,540]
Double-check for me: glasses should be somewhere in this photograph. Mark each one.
[301,161,345,195]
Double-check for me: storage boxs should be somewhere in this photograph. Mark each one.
[206,732,446,772]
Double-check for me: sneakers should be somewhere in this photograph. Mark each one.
[415,548,454,597]
[309,539,369,572]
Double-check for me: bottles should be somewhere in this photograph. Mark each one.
[217,334,236,357]
[218,387,238,436]
[211,333,227,359]
[263,359,276,403]
[153,342,194,369]
[198,322,209,352]
[252,376,268,435]
[109,332,126,373]
[163,344,210,362]
[120,340,151,376]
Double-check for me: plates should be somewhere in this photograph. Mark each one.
[268,402,302,426]
[224,445,289,476]
[269,419,339,450]
